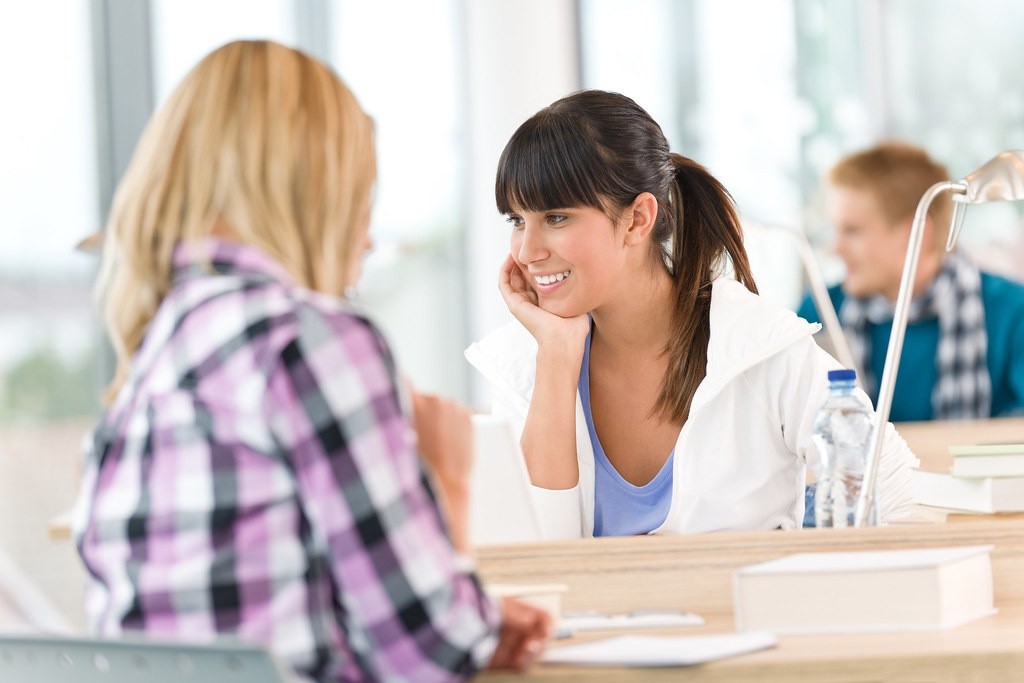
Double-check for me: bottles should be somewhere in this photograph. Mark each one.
[813,369,881,528]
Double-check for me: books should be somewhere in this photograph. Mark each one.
[484,440,1024,667]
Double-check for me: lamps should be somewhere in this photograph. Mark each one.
[854,148,1024,534]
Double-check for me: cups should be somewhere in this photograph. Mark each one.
[469,415,542,546]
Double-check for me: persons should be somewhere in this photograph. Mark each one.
[794,141,1024,422]
[462,91,925,548]
[75,40,550,683]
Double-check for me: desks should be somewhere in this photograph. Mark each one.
[469,525,1024,683]
[886,414,1024,530]
